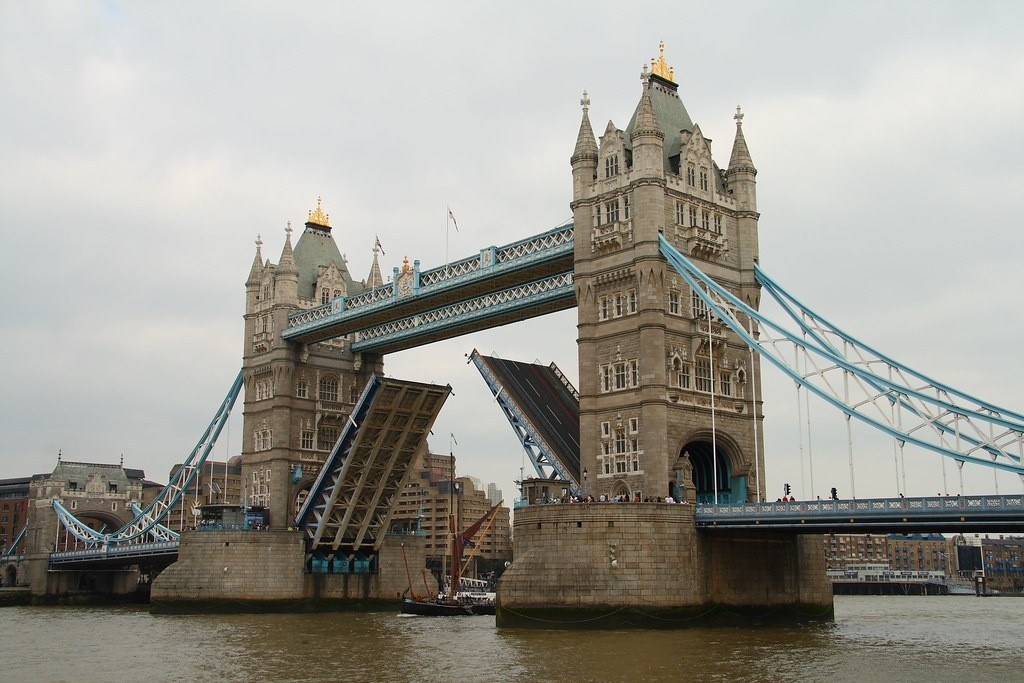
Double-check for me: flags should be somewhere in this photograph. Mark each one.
[376,237,385,255]
[448,207,459,231]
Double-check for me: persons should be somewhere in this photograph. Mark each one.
[122,518,416,547]
[427,591,496,606]
[744,493,961,510]
[538,494,691,506]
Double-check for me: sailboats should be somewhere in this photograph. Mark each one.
[399,531,496,616]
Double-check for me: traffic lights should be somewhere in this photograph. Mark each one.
[785,483,791,495]
[830,488,838,498]
